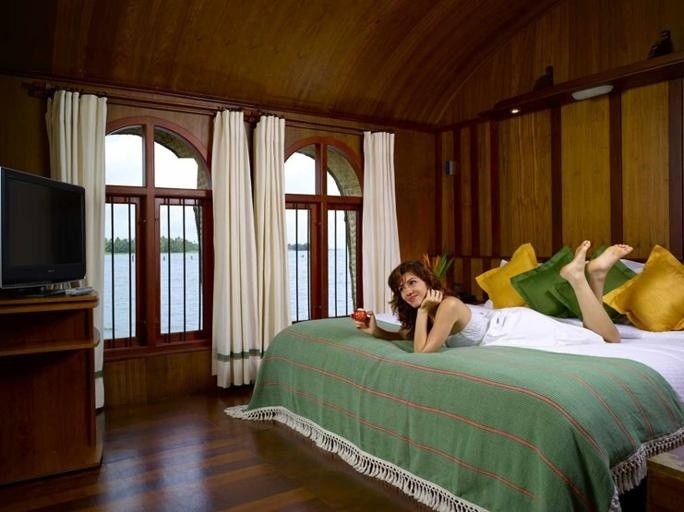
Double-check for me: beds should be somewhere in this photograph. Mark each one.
[225,253,683,512]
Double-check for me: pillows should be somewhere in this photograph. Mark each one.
[546,241,636,324]
[474,242,542,312]
[601,244,684,331]
[509,245,578,319]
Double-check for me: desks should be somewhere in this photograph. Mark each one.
[644,442,683,512]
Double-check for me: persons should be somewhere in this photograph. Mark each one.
[354,240,633,355]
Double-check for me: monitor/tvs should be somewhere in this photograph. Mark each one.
[0,166,86,297]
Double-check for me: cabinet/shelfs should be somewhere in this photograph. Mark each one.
[0,290,106,483]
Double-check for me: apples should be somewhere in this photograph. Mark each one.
[354,311,367,322]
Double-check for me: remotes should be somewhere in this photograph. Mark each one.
[65,286,94,297]
